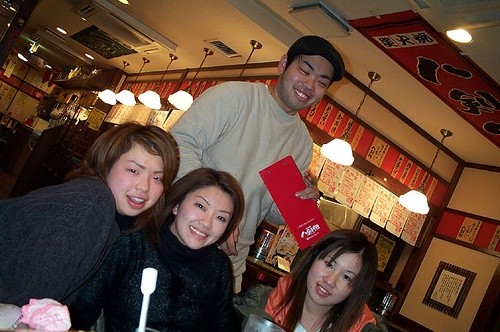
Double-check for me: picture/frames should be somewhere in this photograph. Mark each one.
[422,260,477,319]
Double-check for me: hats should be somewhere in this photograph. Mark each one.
[287,35,345,81]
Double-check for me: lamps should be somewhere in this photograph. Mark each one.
[138,53,178,110]
[32,26,92,66]
[289,1,353,40]
[398,128,452,215]
[98,61,130,106]
[116,57,150,107]
[167,47,214,111]
[237,40,263,81]
[320,72,381,166]
[18,52,32,62]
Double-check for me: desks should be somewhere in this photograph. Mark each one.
[243,255,411,332]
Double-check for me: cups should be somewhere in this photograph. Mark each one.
[243,314,286,332]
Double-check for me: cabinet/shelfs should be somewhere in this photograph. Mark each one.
[259,219,419,320]
[46,67,127,158]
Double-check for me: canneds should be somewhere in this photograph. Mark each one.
[377,292,398,318]
[252,230,275,262]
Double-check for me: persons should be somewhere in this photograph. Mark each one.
[233,228,388,332]
[167,35,345,307]
[0,122,180,308]
[68,167,245,332]
